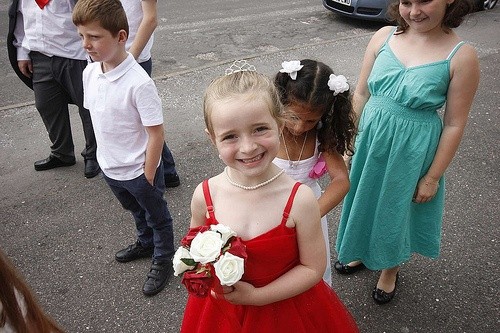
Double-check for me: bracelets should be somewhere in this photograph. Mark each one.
[423,176,441,187]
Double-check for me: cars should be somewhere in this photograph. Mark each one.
[323,0,500,26]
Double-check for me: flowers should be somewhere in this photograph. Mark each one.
[172,223,247,298]
[328,74,350,97]
[309,155,327,178]
[279,59,304,80]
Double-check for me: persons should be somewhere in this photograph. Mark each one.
[171,58,360,333]
[8,0,179,188]
[71,0,173,297]
[334,0,479,304]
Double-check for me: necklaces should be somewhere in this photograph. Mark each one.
[279,127,309,170]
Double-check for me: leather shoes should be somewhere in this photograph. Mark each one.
[334,258,366,273]
[116,239,153,262]
[163,173,181,188]
[34,154,74,171]
[373,271,398,304]
[84,158,100,178]
[142,258,174,296]
